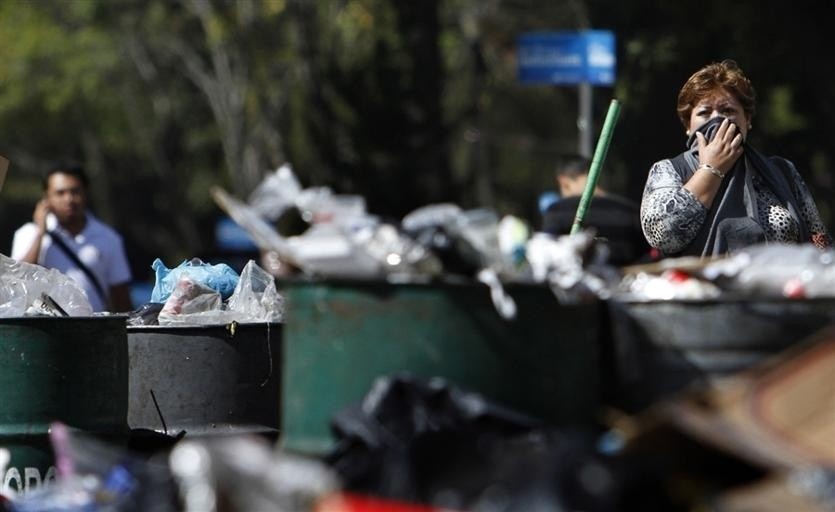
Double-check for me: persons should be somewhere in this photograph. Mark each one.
[12,166,132,315]
[542,154,651,271]
[639,59,832,259]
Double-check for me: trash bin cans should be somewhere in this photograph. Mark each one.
[127,316,286,493]
[608,286,835,496]
[0,311,135,490]
[278,276,604,496]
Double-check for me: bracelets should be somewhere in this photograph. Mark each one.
[699,164,724,182]
